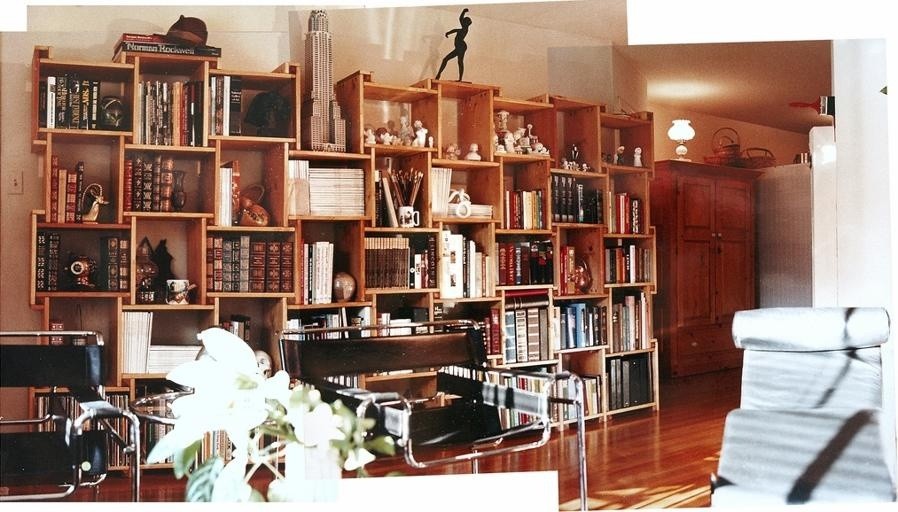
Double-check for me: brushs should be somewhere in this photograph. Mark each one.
[787,95,835,117]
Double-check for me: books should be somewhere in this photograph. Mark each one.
[38,70,101,130]
[123,154,175,212]
[35,233,128,292]
[219,314,251,343]
[138,79,242,147]
[135,387,232,465]
[206,236,293,293]
[215,159,240,226]
[499,174,653,430]
[50,323,90,346]
[289,160,365,215]
[375,169,492,229]
[50,156,84,222]
[121,311,204,374]
[434,304,501,384]
[301,242,334,304]
[286,305,430,391]
[110,34,222,63]
[37,392,131,466]
[364,231,497,298]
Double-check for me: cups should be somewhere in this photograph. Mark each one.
[165,280,190,305]
[398,205,420,228]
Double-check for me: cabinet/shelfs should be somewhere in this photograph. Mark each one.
[29,47,658,468]
[653,161,759,378]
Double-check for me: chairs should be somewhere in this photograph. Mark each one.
[0,325,144,501]
[280,321,589,512]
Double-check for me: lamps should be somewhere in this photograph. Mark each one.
[668,120,695,162]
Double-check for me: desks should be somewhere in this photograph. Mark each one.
[129,385,287,470]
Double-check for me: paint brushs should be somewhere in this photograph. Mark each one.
[388,167,424,207]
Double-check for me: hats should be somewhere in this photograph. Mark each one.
[154,15,215,49]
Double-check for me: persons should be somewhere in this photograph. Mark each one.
[435,9,472,82]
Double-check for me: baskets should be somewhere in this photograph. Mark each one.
[703,127,775,168]
